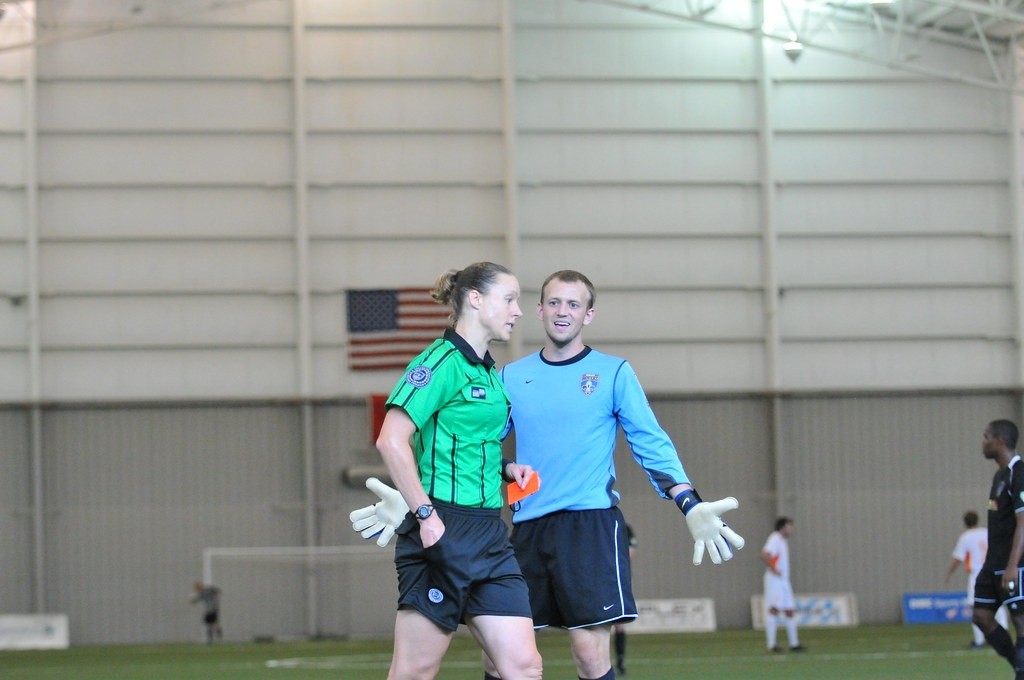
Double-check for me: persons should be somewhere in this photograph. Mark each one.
[973,419,1024,680]
[944,510,1010,648]
[376,261,543,680]
[761,517,807,651]
[349,270,745,680]
[614,522,637,674]
[189,580,223,644]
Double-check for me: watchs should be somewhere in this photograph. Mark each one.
[414,504,434,520]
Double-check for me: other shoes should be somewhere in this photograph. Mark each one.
[768,645,784,654]
[968,642,983,650]
[791,644,807,650]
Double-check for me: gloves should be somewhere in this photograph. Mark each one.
[674,488,745,566]
[349,478,410,546]
